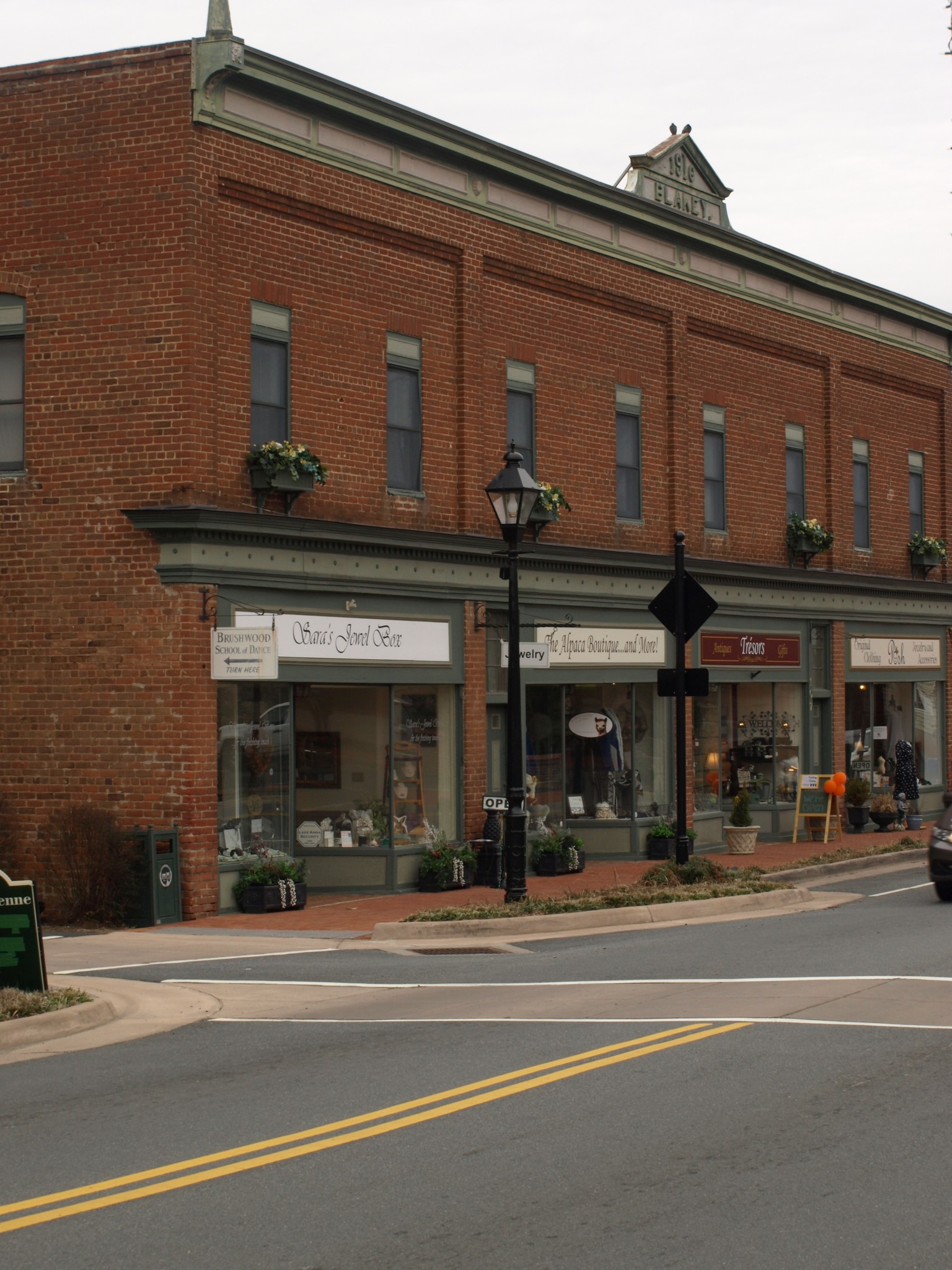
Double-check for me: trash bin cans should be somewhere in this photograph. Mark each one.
[112,824,184,926]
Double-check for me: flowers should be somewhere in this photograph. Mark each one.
[786,511,833,553]
[349,808,373,830]
[908,531,947,558]
[420,819,476,888]
[530,817,583,870]
[247,439,329,486]
[232,834,309,899]
[537,481,571,520]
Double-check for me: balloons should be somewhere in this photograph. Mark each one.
[707,771,719,795]
[824,780,836,793]
[833,784,845,796]
[834,772,846,785]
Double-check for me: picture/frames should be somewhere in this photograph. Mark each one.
[296,731,341,789]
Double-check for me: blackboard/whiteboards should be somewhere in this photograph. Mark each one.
[795,774,841,817]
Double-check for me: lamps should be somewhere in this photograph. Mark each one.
[705,753,723,770]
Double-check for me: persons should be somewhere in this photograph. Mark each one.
[894,740,926,829]
[884,705,903,762]
[401,761,415,778]
[879,756,896,786]
[894,792,908,831]
[393,782,408,800]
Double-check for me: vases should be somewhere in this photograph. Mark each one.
[792,532,820,554]
[529,502,559,522]
[243,882,307,910]
[356,825,371,837]
[537,851,585,874]
[869,812,923,832]
[912,552,939,565]
[250,465,317,492]
[419,860,475,887]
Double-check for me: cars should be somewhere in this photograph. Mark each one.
[928,791,952,901]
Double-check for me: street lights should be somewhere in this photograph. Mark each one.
[478,436,548,904]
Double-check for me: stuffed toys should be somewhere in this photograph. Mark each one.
[526,773,549,834]
[595,801,617,818]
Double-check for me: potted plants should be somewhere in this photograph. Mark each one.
[647,813,697,856]
[844,777,872,833]
[723,791,760,855]
[353,796,389,840]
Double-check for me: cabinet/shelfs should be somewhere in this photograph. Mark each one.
[381,740,428,838]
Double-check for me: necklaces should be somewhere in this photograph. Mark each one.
[407,769,412,774]
[397,788,404,796]
